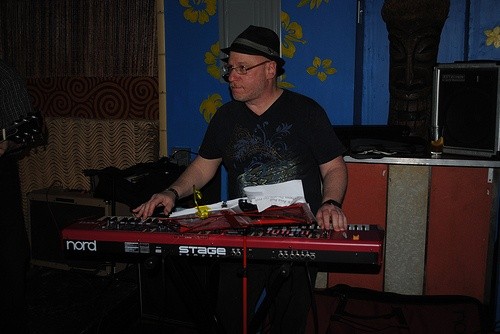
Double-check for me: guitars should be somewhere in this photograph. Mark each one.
[0,110,48,154]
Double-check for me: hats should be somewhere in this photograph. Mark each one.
[220,25,285,68]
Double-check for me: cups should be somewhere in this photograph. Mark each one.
[430,125,445,154]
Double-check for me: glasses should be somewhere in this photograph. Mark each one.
[222,61,271,76]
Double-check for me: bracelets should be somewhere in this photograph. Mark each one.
[318,199,343,210]
[161,186,179,202]
[132,24,349,334]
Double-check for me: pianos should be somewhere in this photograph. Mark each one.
[61,216,385,275]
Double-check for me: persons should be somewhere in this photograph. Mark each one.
[0,61,35,334]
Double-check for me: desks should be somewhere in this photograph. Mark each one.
[324,156,500,298]
